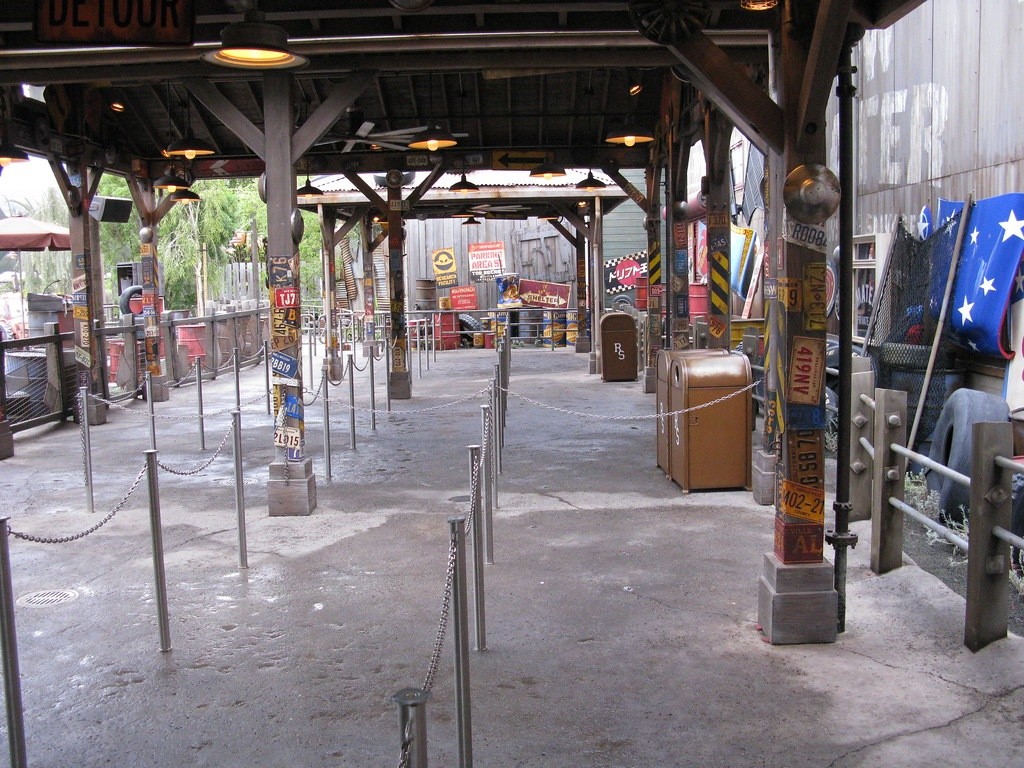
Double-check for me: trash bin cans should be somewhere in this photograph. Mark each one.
[600,313,639,381]
[670,353,752,495]
[654,347,729,479]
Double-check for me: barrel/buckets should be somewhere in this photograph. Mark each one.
[566,309,591,347]
[414,278,437,310]
[27,291,72,346]
[518,307,543,347]
[433,311,461,350]
[543,308,566,347]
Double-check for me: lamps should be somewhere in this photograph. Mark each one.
[605,65,655,146]
[528,75,566,180]
[538,203,558,219]
[153,78,215,204]
[200,0,310,72]
[451,205,474,217]
[296,98,324,199]
[450,99,479,195]
[0,110,29,166]
[408,72,458,151]
[575,87,606,191]
[461,216,481,225]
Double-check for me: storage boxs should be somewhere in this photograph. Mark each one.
[117,261,166,297]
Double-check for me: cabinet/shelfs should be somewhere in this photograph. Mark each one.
[852,233,892,347]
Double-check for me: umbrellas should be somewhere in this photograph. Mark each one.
[0,216,72,338]
